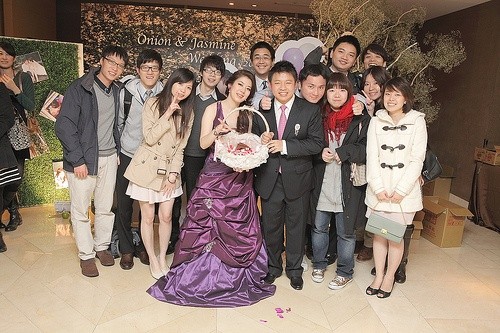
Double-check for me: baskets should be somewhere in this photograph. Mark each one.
[212,105,270,173]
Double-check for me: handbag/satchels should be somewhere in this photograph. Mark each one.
[25,117,49,160]
[7,121,31,151]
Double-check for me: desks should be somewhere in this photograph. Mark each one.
[467,161,500,233]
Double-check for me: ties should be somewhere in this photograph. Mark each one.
[278,105,288,139]
[262,81,268,90]
[145,90,153,101]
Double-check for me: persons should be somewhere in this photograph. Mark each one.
[365,77,428,297]
[166,41,325,290]
[55,46,129,277]
[21,57,67,186]
[119,49,164,269]
[146,69,277,308]
[303,34,424,289]
[124,68,197,280]
[0,41,36,252]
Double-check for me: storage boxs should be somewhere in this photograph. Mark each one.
[411,165,474,248]
[473,143,500,166]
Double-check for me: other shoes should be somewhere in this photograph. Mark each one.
[149,266,164,280]
[377,276,395,298]
[161,268,170,275]
[0,232,7,252]
[366,285,379,295]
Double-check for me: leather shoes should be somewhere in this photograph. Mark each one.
[356,246,373,261]
[80,258,99,277]
[354,241,364,254]
[120,252,135,270]
[290,277,303,290]
[96,249,115,266]
[325,252,337,265]
[135,250,151,265]
[264,272,277,284]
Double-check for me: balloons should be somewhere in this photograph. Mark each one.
[283,49,304,74]
[300,43,315,57]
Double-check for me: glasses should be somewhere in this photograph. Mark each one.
[103,56,125,70]
[203,68,222,76]
[137,65,160,72]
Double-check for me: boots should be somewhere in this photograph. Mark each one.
[0,190,22,231]
[394,224,414,283]
[371,252,389,276]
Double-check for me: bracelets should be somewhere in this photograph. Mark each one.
[214,128,217,136]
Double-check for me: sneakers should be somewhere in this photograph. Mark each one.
[328,275,354,290]
[312,268,324,283]
[301,255,309,272]
